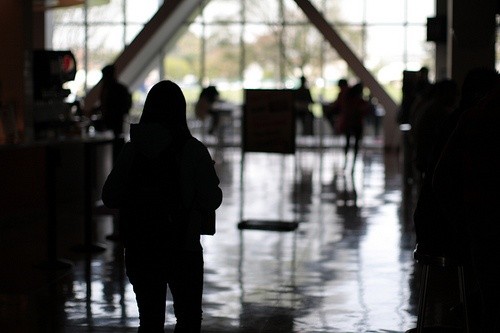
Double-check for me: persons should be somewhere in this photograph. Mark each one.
[295,75,371,156]
[419,67,430,77]
[90,64,223,333]
